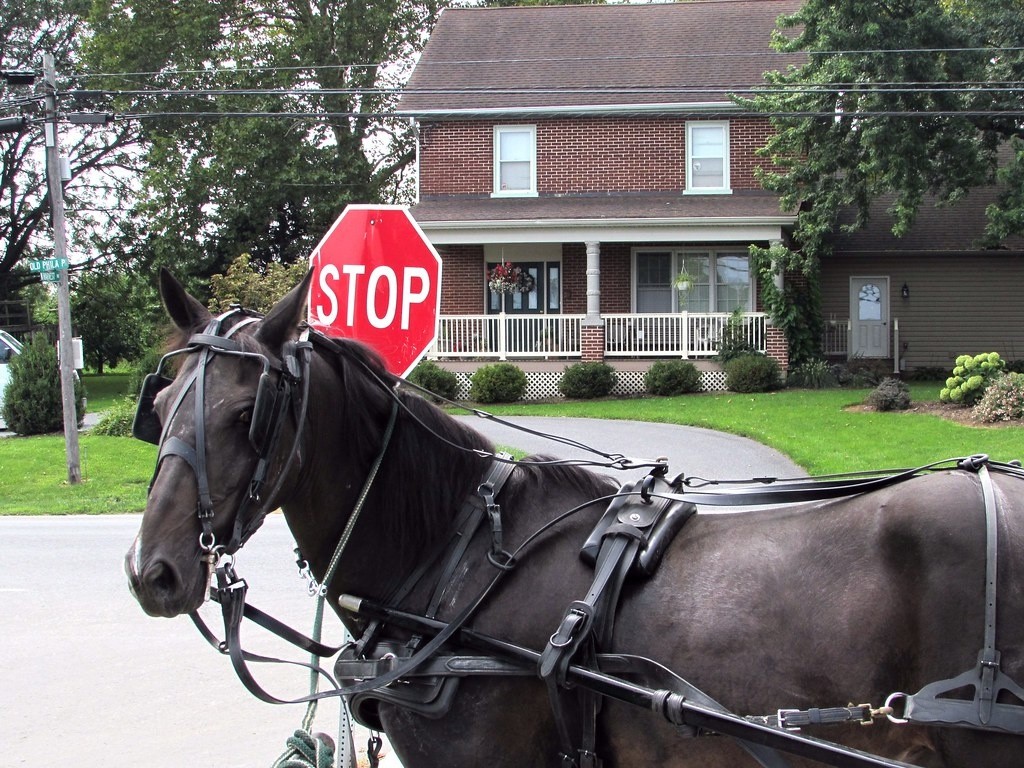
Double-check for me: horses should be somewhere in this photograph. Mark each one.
[122,266,1024,767]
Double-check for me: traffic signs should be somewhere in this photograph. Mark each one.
[28,258,69,282]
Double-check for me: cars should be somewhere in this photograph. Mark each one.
[0,329,86,431]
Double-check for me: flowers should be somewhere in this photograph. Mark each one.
[489,261,520,293]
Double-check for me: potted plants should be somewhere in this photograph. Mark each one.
[672,274,695,308]
[541,326,554,345]
[438,327,453,352]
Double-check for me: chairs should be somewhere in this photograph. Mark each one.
[605,325,626,350]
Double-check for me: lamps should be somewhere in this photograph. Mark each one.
[902,283,909,297]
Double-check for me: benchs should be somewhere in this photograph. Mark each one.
[625,322,711,358]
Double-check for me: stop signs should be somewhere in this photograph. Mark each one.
[308,202,441,390]
